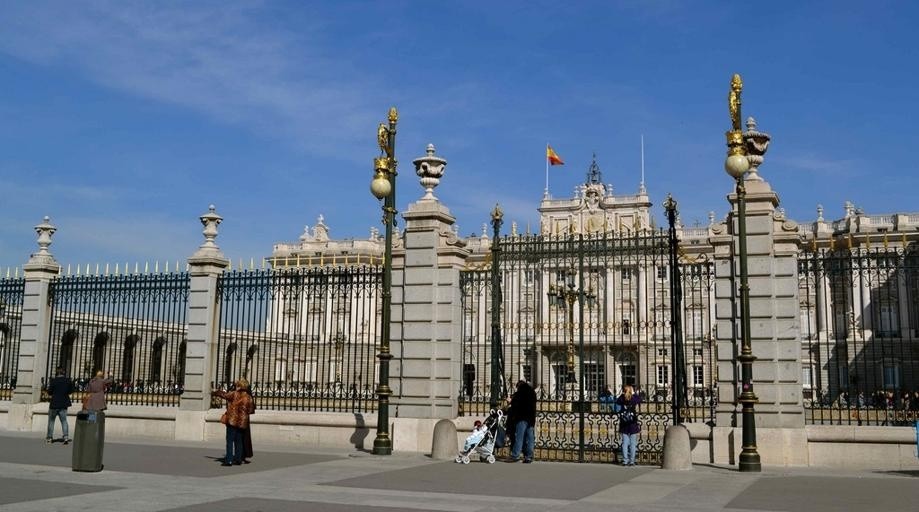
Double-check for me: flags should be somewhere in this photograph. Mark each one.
[547,145,564,166]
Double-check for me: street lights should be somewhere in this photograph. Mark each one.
[722,71,763,473]
[549,267,597,411]
[334,327,345,382]
[368,104,401,456]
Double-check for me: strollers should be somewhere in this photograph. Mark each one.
[455,408,504,464]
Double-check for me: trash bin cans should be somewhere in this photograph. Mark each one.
[71,410,105,472]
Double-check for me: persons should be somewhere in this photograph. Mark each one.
[238,389,255,464]
[83,368,113,412]
[43,367,75,444]
[617,385,642,467]
[504,381,536,463]
[214,378,253,467]
[473,420,481,435]
